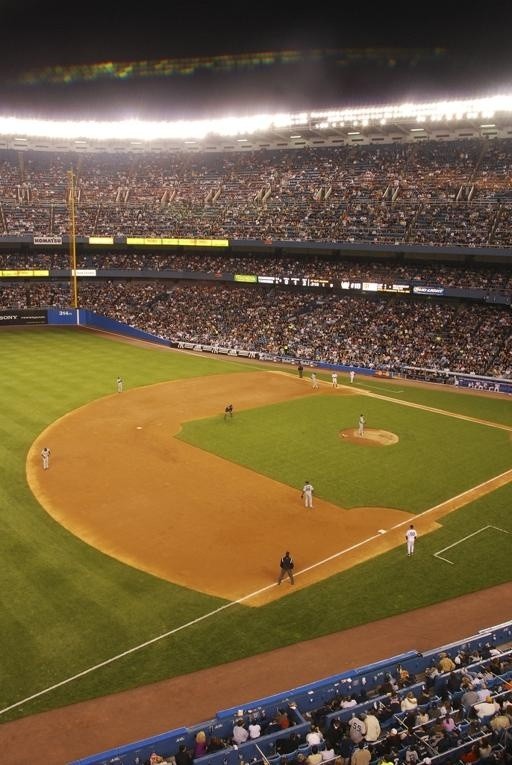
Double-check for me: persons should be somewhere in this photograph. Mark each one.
[277,551,294,585]
[301,481,314,508]
[358,413,367,435]
[41,448,51,469]
[144,645,512,765]
[405,525,417,557]
[224,403,232,419]
[0,135,512,393]
